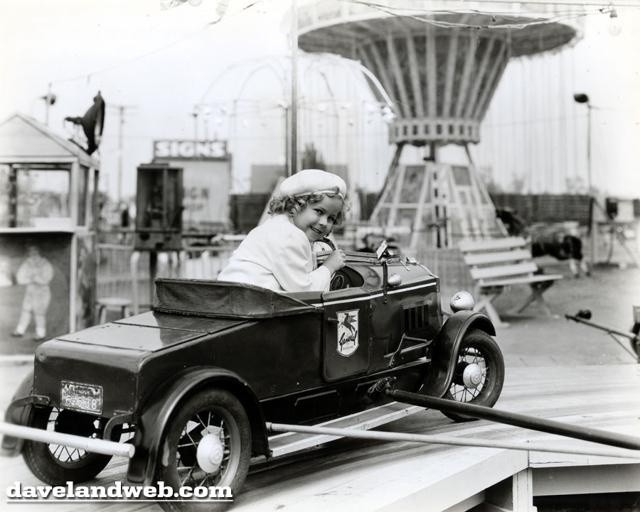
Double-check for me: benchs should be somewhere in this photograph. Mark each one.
[452,234,565,330]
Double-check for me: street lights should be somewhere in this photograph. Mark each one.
[573,92,595,268]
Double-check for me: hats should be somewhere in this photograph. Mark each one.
[280,169,346,201]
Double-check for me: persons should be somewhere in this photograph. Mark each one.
[217,168,348,292]
[10,245,55,341]
[531,229,590,279]
